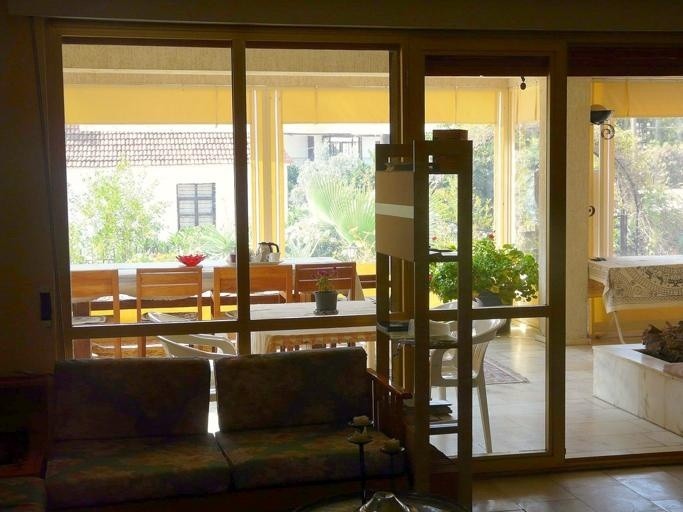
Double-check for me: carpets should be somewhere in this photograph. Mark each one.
[431,356,528,388]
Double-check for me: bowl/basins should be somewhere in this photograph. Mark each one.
[177,255,207,266]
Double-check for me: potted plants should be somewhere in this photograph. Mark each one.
[428,239,538,336]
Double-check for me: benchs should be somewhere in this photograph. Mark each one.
[44,347,412,509]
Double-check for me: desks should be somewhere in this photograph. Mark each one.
[587,254,683,343]
[250,300,378,354]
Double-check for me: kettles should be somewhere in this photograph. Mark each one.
[257,242,278,261]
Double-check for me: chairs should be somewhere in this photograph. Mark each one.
[429,300,507,453]
[68,260,358,401]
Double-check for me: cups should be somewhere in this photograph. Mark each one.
[268,252,280,262]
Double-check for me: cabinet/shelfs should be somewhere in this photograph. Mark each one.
[373,138,474,511]
